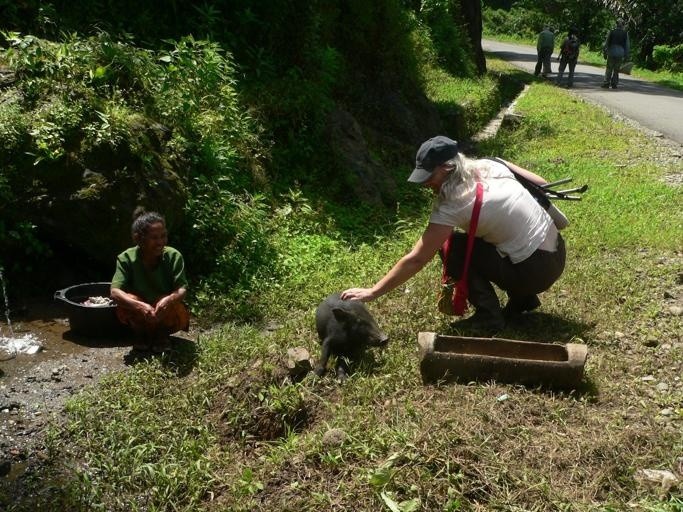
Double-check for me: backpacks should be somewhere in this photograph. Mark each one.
[564,35,578,60]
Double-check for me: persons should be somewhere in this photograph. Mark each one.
[600,18,632,89]
[338,133,572,331]
[110,206,193,357]
[553,28,581,87]
[533,24,555,78]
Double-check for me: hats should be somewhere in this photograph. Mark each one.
[408,136,458,184]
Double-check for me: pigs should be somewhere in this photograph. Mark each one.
[315,292,389,380]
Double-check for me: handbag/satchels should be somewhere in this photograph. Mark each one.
[450,307,513,331]
[512,170,570,229]
[438,276,468,316]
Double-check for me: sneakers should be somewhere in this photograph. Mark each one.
[601,83,617,89]
[134,338,172,352]
[503,291,541,315]
